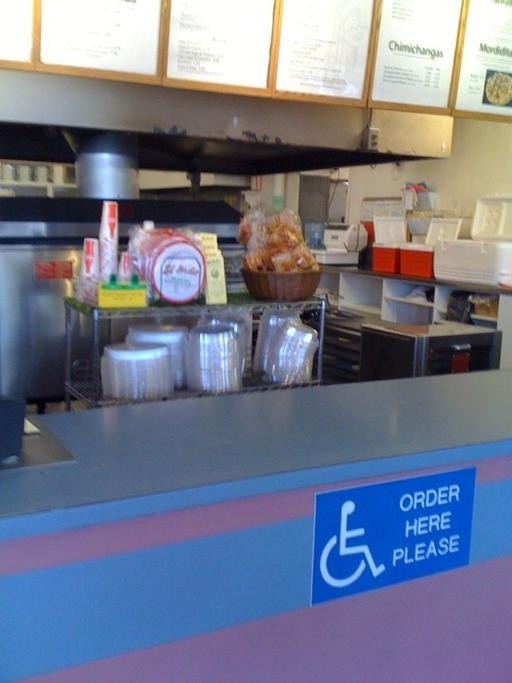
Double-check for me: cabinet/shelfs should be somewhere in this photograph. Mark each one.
[302,256,511,383]
[62,296,327,411]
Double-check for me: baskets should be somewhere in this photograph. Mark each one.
[240,268,322,303]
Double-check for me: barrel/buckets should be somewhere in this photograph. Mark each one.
[304,222,325,249]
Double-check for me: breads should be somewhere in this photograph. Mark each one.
[239,223,319,272]
[474,299,499,318]
